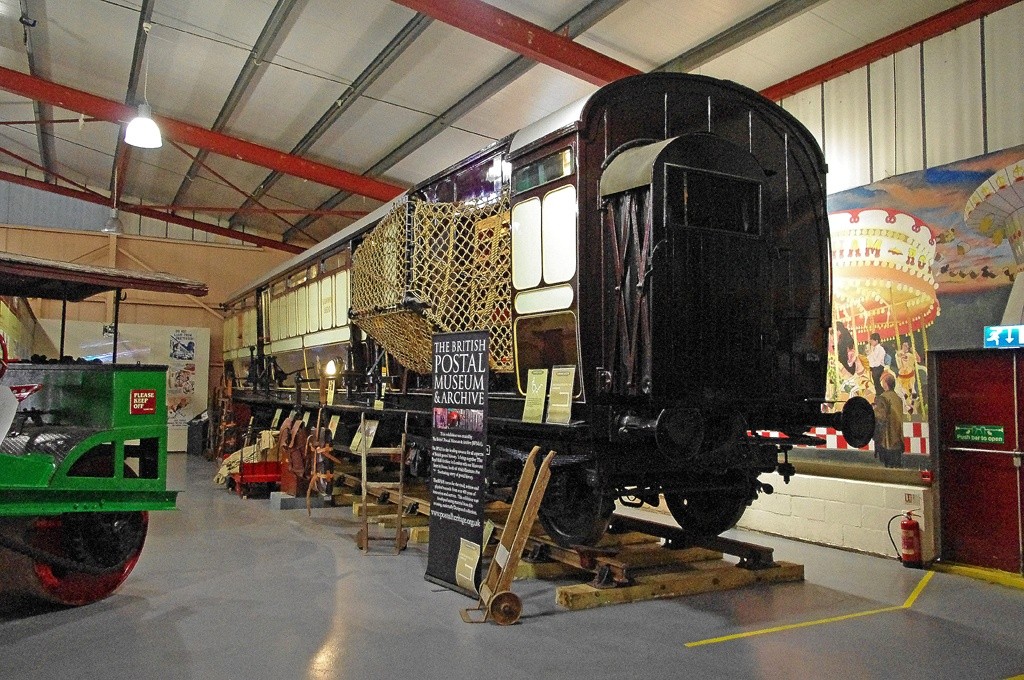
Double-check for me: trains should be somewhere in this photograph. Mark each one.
[220,72,835,571]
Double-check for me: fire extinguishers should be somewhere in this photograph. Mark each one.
[887,508,921,568]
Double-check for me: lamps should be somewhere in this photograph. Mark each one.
[121,21,165,150]
